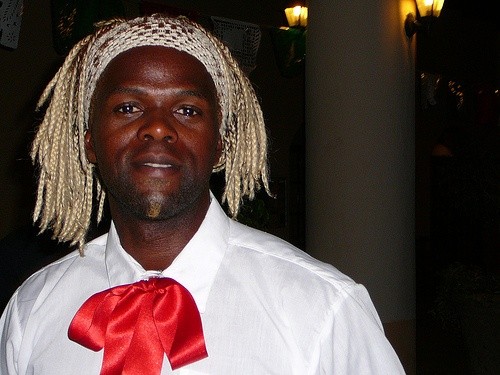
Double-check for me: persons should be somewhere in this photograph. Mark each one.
[0,11,408,374]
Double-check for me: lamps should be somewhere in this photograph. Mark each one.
[282,1,308,69]
[406,0,446,38]
